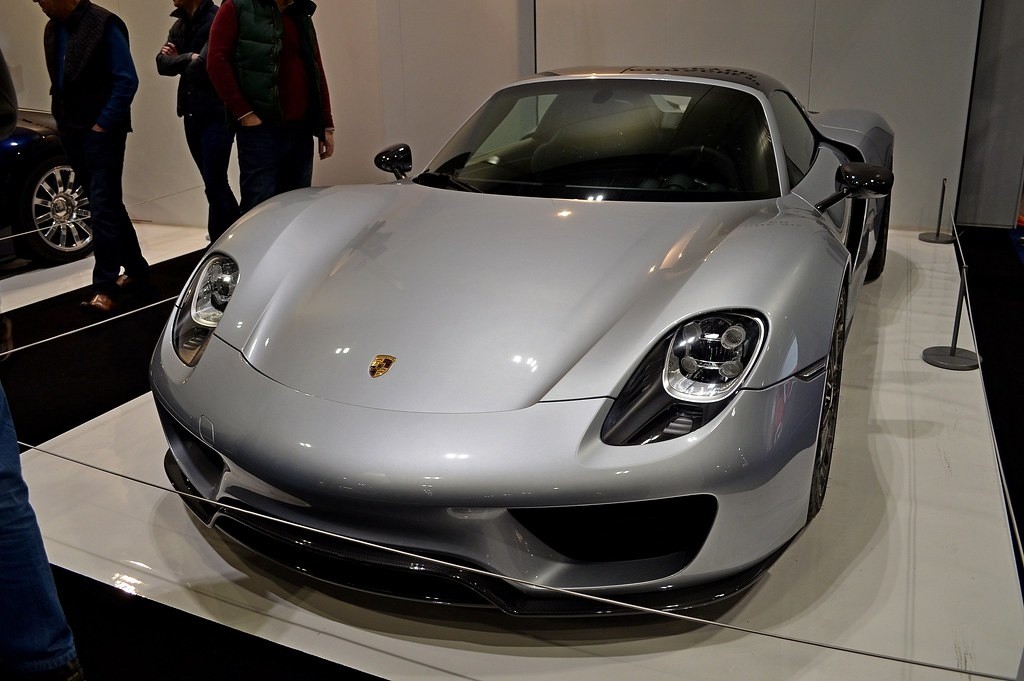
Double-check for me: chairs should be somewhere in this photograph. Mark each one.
[668,88,770,196]
[531,97,656,185]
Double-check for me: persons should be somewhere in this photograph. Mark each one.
[206,0,335,215]
[0,47,18,143]
[31,0,150,313]
[156,0,237,250]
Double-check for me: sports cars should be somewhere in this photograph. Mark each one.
[138,68,896,629]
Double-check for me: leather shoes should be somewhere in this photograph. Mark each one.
[116,263,149,287]
[81,294,114,311]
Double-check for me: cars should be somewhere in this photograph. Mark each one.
[0,108,93,267]
